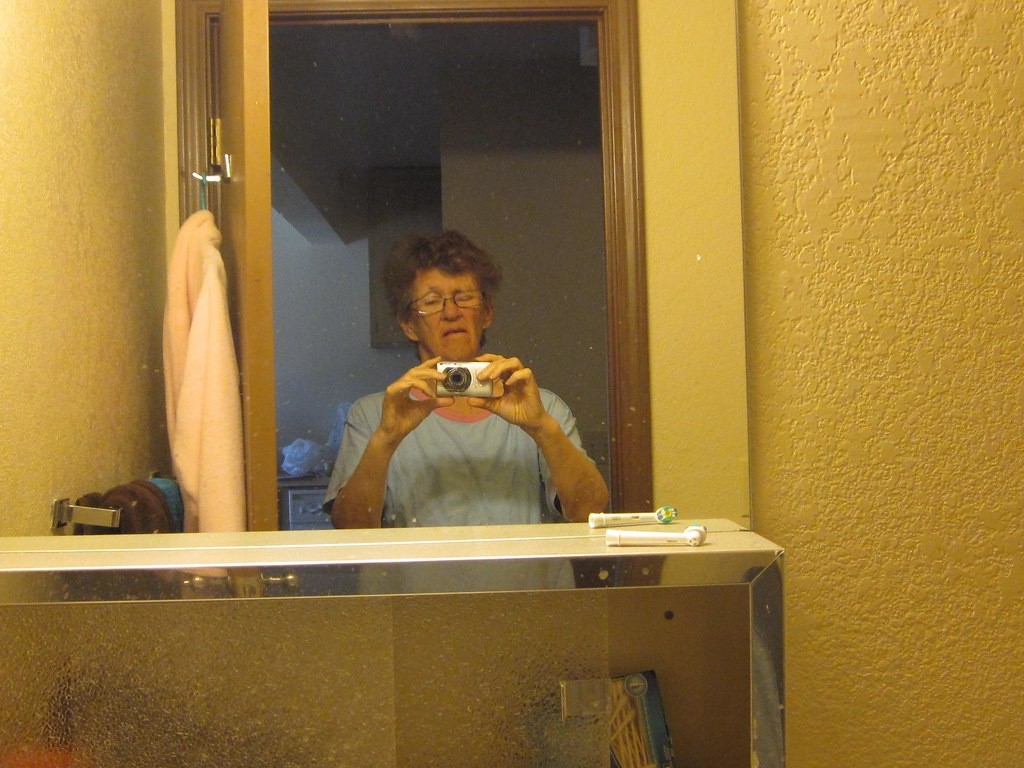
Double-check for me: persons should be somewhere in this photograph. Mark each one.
[321,230,609,596]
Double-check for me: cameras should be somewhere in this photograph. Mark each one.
[436,361,493,398]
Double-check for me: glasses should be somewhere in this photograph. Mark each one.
[407,289,487,314]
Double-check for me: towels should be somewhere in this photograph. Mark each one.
[161,208,248,533]
[74,478,183,535]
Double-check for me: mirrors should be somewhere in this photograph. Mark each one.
[203,0,657,532]
